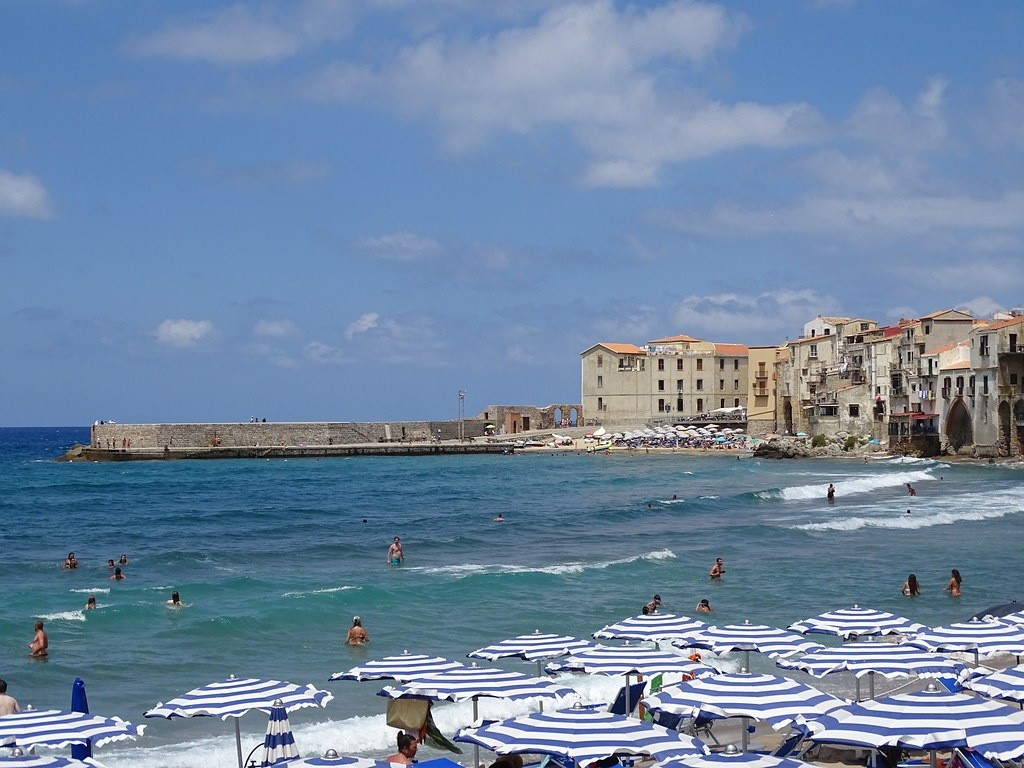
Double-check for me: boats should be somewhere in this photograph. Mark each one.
[796,431,809,438]
[583,431,594,443]
[600,433,611,441]
[526,440,546,447]
[612,432,623,444]
[505,440,526,448]
[554,436,572,444]
[622,424,747,448]
[593,426,606,439]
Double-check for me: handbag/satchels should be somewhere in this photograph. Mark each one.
[386,697,428,730]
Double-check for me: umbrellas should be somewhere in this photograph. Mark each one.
[485,425,495,433]
[144,674,335,768]
[263,749,413,768]
[0,678,147,768]
[593,424,747,446]
[329,604,1024,768]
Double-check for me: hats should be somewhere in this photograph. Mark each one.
[353,616,361,623]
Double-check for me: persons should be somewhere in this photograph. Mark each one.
[664,437,713,449]
[828,484,835,498]
[250,416,266,423]
[217,437,220,447]
[28,621,48,657]
[907,484,915,496]
[696,413,699,421]
[345,616,368,645]
[920,421,924,435]
[385,730,418,765]
[497,753,523,768]
[170,436,173,446]
[107,438,132,449]
[496,513,504,521]
[0,680,20,717]
[642,558,726,617]
[63,552,183,610]
[387,537,405,566]
[902,569,962,595]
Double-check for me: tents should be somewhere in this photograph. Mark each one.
[709,407,747,421]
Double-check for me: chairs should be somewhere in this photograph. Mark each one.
[607,679,1024,768]
